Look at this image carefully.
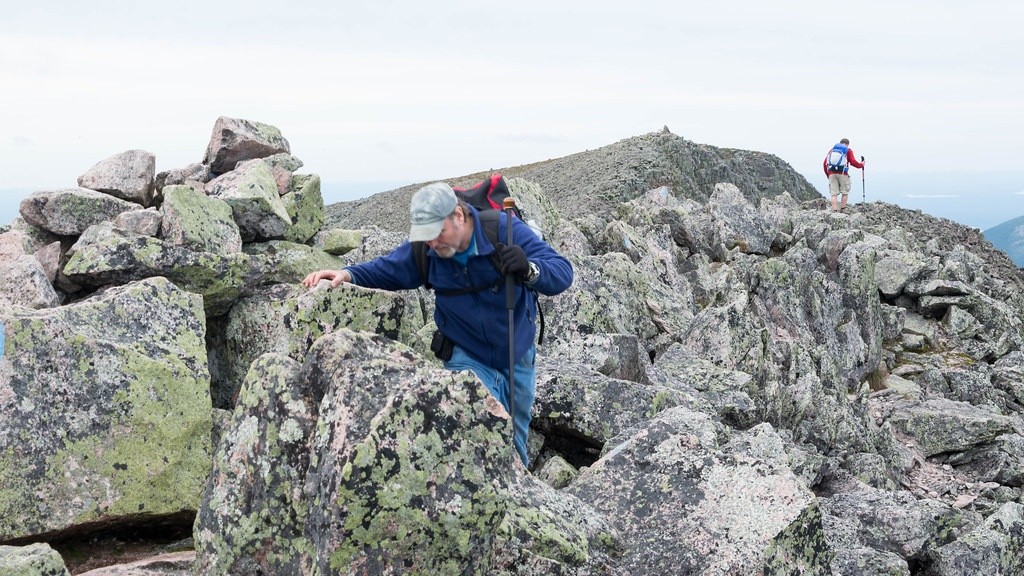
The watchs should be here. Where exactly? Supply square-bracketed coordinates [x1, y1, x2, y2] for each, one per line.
[529, 263, 540, 280]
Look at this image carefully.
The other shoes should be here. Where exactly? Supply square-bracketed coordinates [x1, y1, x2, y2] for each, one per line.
[840, 207, 851, 215]
[833, 209, 838, 213]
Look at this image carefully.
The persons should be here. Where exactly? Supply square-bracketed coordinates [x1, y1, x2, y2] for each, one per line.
[823, 138, 864, 212]
[303, 182, 574, 467]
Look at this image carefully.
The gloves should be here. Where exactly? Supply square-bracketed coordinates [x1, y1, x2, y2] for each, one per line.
[495, 242, 530, 280]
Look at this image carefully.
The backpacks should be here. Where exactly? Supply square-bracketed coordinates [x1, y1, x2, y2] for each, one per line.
[826, 142, 849, 172]
[411, 174, 526, 290]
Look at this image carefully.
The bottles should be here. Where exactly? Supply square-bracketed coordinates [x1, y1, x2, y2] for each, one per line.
[527, 219, 543, 242]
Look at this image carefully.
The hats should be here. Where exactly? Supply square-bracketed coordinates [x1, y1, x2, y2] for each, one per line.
[408, 183, 458, 242]
